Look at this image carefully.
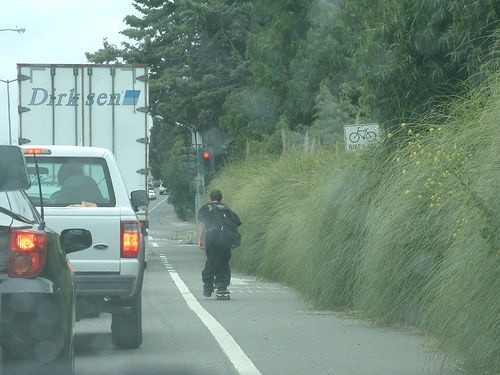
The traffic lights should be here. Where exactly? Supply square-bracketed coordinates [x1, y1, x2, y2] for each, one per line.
[198, 146, 214, 175]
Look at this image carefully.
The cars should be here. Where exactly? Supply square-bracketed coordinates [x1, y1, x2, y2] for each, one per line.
[148, 179, 168, 195]
[149, 190, 156, 200]
[0, 191, 93, 375]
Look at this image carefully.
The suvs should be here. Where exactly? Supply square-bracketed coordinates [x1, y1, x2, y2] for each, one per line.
[12, 144, 150, 350]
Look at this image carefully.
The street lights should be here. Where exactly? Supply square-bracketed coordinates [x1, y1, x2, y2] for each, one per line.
[0, 27, 27, 36]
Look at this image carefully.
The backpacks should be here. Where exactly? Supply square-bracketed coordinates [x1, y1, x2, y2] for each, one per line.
[207, 202, 241, 247]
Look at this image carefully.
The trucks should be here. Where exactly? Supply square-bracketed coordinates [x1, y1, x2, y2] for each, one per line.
[17, 62, 150, 239]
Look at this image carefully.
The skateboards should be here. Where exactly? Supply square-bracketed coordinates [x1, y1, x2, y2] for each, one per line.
[216, 290, 231, 301]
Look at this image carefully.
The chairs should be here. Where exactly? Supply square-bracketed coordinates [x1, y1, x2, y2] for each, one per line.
[55, 176, 104, 204]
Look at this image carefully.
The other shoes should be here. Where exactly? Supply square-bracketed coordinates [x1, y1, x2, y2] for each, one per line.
[216, 289, 230, 300]
[203, 284, 213, 297]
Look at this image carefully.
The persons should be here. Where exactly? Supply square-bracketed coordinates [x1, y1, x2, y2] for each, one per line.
[198, 189, 242, 301]
[46, 161, 85, 204]
